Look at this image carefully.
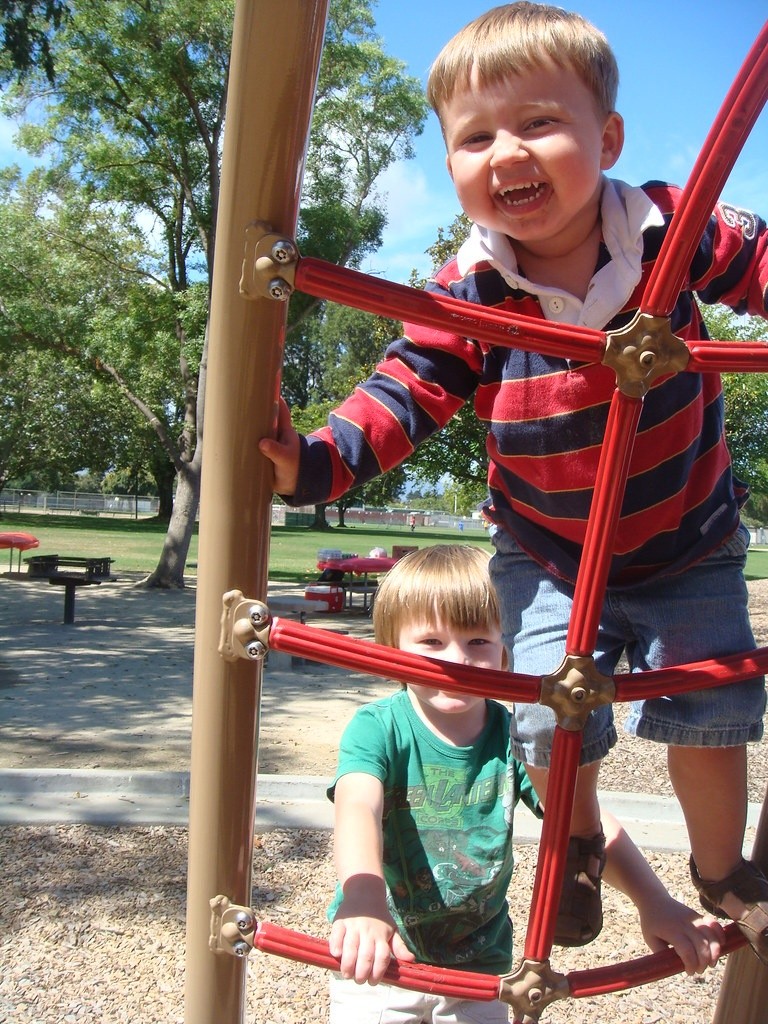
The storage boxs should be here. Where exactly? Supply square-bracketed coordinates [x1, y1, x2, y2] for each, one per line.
[305, 584, 344, 612]
[392, 545, 419, 560]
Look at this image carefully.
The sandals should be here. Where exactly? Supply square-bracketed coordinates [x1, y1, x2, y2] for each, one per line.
[690, 852, 768, 967]
[552, 825, 605, 946]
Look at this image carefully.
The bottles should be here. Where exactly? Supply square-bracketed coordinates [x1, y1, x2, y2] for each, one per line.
[317, 548, 343, 563]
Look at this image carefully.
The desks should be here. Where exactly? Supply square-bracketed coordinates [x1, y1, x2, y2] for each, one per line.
[266, 597, 330, 668]
[318, 558, 398, 614]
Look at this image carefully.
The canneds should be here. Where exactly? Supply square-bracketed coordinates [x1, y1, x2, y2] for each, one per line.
[342, 553, 358, 558]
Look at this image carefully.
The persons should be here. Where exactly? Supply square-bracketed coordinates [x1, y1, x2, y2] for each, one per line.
[458, 520, 464, 532]
[410, 516, 415, 531]
[483, 520, 489, 534]
[258, 0, 768, 966]
[325, 544, 727, 1024]
